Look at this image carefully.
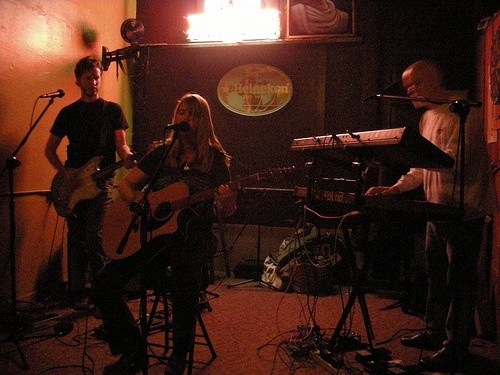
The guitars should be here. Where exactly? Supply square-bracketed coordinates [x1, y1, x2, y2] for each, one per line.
[51, 140, 164, 218]
[102, 165, 302, 261]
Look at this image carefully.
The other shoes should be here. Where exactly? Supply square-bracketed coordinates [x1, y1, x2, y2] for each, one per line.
[102, 349, 150, 375]
[164, 335, 187, 375]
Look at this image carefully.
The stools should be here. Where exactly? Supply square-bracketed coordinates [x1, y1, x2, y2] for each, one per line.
[147, 289, 217, 375]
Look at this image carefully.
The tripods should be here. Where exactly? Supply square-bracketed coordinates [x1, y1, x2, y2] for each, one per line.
[0, 100, 60, 370]
[227, 192, 278, 291]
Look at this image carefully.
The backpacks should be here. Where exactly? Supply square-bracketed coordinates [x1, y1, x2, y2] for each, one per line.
[261, 223, 348, 296]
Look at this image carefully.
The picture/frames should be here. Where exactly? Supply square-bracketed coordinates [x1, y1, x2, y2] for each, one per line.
[286, 0, 355, 39]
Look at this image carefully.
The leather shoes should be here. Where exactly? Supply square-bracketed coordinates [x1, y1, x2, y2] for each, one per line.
[400, 331, 452, 347]
[418, 345, 472, 371]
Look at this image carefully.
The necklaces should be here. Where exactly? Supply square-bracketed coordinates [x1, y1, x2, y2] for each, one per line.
[179, 153, 195, 171]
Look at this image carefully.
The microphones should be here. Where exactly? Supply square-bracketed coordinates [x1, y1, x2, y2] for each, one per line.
[164, 121, 189, 131]
[39, 89, 65, 99]
[363, 83, 401, 104]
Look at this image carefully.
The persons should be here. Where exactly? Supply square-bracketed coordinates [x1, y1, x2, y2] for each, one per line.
[45, 58, 139, 314]
[91, 93, 242, 375]
[289, 0, 349, 36]
[364, 61, 498, 364]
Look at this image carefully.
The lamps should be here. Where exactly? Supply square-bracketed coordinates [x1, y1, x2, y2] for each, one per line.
[102, 19, 155, 76]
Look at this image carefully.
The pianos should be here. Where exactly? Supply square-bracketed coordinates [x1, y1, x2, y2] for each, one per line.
[237, 126, 464, 375]
